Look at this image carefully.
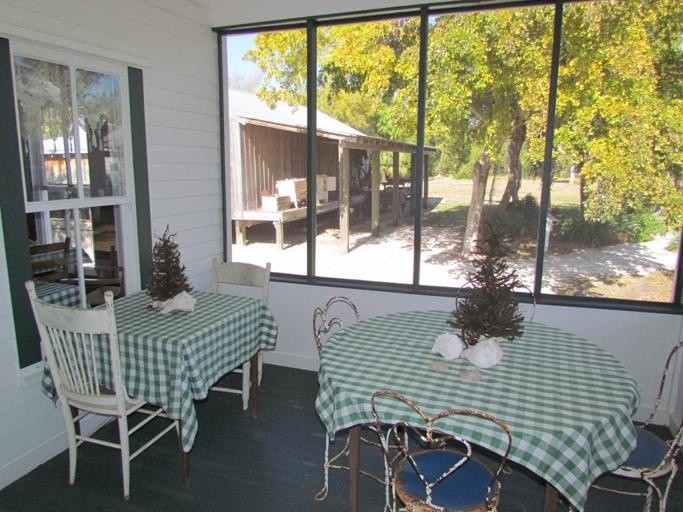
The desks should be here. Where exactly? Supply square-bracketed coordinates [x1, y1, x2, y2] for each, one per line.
[313, 310, 640, 512]
[30, 244, 92, 274]
[40, 289, 277, 488]
[35, 281, 81, 307]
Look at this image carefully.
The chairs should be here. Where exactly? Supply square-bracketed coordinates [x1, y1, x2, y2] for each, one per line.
[591, 341, 683, 511]
[25, 280, 180, 501]
[30, 237, 70, 282]
[86, 267, 124, 305]
[209, 257, 271, 411]
[313, 296, 407, 502]
[371, 389, 512, 511]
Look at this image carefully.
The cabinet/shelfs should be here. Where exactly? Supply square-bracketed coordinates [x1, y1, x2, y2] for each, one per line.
[276, 174, 337, 208]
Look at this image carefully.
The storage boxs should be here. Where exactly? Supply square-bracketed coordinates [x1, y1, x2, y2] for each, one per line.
[262, 194, 290, 211]
